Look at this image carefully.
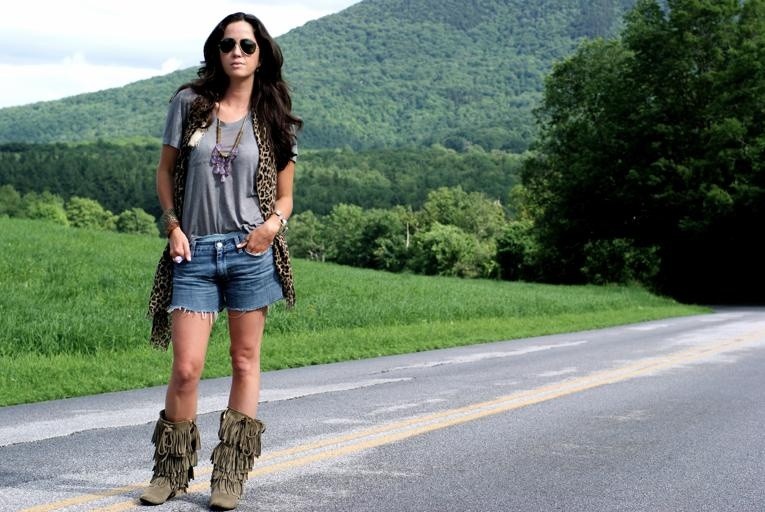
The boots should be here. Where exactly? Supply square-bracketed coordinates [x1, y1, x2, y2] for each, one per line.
[139, 408, 201, 507]
[207, 408, 264, 511]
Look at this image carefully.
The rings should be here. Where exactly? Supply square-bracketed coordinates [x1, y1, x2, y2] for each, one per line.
[174, 256, 182, 264]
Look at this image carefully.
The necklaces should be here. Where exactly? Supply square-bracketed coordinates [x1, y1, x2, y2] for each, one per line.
[209, 104, 246, 183]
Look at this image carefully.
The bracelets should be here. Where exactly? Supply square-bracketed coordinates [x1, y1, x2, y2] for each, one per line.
[274, 211, 288, 226]
[159, 208, 176, 237]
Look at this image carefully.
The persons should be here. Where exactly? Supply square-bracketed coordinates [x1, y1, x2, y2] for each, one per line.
[140, 10, 299, 511]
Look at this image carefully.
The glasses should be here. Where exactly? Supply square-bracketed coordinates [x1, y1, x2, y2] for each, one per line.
[218, 37, 258, 56]
